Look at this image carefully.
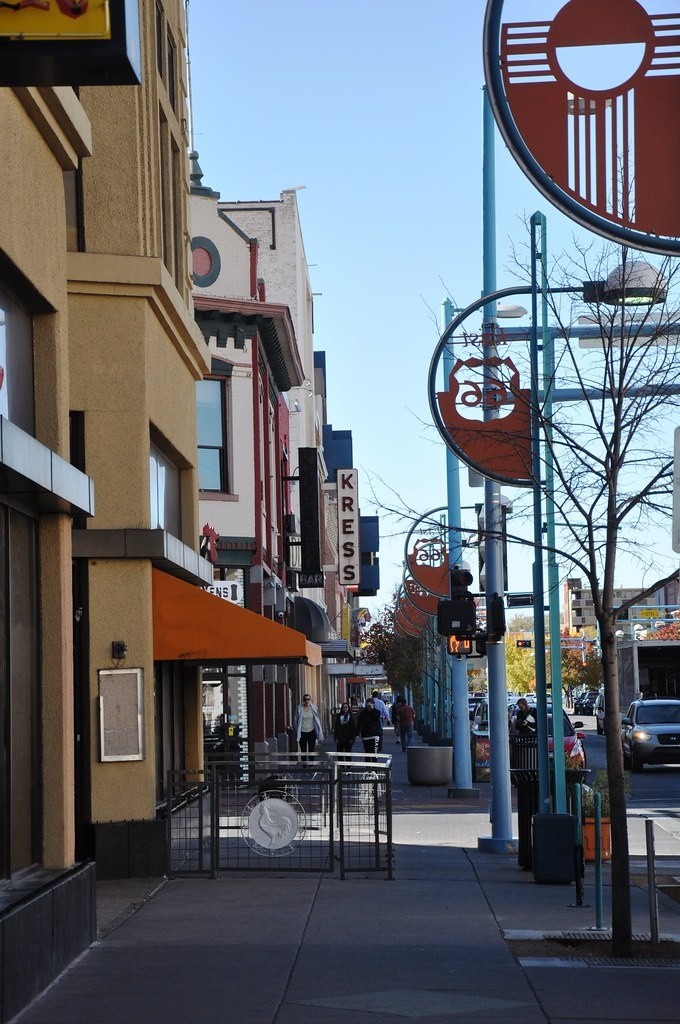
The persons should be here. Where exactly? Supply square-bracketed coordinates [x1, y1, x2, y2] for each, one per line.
[371, 691, 415, 752]
[516, 698, 537, 734]
[356, 698, 382, 773]
[334, 703, 356, 775]
[665, 608, 674, 628]
[294, 694, 324, 776]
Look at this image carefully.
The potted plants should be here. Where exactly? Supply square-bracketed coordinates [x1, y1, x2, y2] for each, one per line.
[556, 751, 631, 861]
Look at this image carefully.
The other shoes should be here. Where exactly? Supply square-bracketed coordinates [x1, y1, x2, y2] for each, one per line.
[396, 737, 400, 744]
[303, 767, 309, 776]
[402, 748, 407, 752]
[310, 767, 315, 777]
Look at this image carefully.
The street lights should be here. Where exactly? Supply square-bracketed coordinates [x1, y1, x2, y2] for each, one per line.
[404, 495, 515, 789]
[443, 298, 527, 794]
[427, 212, 669, 813]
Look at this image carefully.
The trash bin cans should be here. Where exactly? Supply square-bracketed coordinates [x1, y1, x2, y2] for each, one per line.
[509, 733, 538, 780]
[508, 768, 591, 869]
[531, 813, 576, 884]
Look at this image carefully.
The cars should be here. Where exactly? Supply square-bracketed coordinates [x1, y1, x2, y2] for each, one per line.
[621, 696, 680, 773]
[432, 686, 605, 785]
[382, 690, 393, 704]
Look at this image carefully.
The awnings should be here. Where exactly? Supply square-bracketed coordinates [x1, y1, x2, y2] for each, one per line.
[317, 640, 387, 684]
[295, 596, 332, 643]
[152, 566, 323, 668]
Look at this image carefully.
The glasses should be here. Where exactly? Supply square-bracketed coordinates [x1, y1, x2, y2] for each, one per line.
[365, 704, 372, 707]
[303, 698, 309, 701]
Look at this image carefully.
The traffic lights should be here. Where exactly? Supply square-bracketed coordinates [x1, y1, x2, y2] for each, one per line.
[516, 640, 532, 648]
[451, 570, 474, 604]
[446, 633, 473, 655]
